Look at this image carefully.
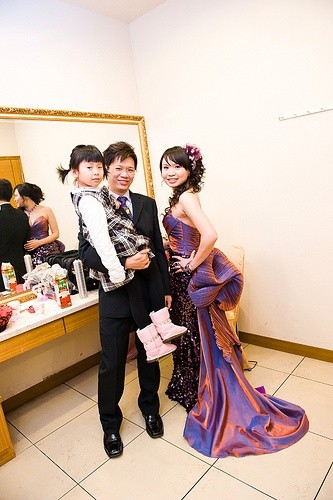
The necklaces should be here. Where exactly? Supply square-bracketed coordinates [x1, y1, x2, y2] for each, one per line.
[24, 204, 37, 212]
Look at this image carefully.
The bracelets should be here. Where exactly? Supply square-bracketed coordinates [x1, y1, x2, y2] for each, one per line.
[185, 261, 191, 272]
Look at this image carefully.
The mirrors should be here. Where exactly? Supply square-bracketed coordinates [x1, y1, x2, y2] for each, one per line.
[0, 107, 155, 307]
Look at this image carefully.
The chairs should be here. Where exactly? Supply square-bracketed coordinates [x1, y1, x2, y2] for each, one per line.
[225, 244, 244, 333]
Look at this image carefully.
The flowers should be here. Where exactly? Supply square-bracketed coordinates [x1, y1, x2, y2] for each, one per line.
[183, 143, 202, 170]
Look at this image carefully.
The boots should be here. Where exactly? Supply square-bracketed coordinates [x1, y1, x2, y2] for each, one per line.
[136, 323, 177, 362]
[150, 306, 187, 343]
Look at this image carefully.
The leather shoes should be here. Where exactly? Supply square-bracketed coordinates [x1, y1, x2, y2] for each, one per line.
[143, 412, 164, 438]
[103, 431, 123, 458]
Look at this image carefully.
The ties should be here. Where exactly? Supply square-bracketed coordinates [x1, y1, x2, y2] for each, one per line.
[117, 197, 133, 221]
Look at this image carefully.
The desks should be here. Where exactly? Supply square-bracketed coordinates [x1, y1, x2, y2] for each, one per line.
[0, 291, 99, 466]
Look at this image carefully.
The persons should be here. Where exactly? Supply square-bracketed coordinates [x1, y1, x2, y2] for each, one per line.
[0, 178, 32, 293]
[14, 182, 65, 268]
[58, 145, 188, 363]
[77, 141, 172, 458]
[160, 146, 309, 458]
